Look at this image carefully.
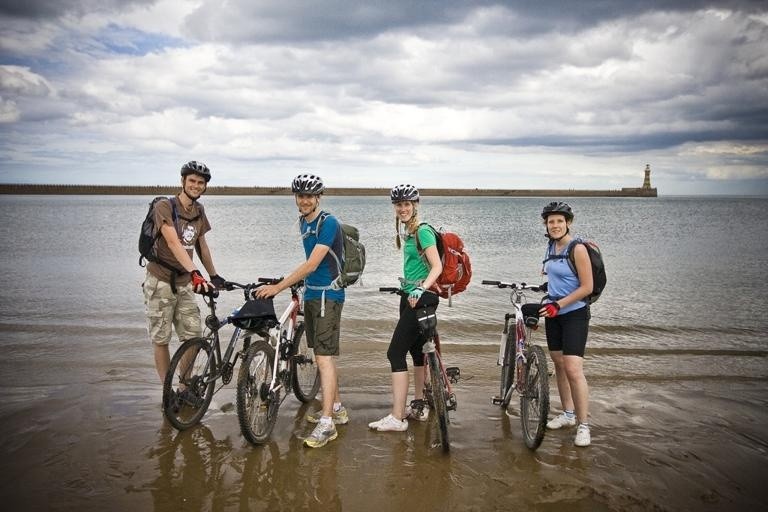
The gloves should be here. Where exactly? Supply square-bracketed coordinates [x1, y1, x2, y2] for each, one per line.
[191, 269, 217, 295]
[211, 274, 227, 292]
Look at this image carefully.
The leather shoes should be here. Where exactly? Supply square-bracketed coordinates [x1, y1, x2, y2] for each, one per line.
[170, 389, 180, 413]
[177, 387, 204, 409]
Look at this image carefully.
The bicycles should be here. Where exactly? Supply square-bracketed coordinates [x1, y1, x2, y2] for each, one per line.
[235, 279, 326, 446]
[380, 285, 460, 452]
[482, 279, 561, 451]
[162, 277, 281, 432]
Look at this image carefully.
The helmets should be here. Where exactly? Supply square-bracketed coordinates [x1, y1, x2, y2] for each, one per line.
[292, 174, 325, 196]
[180, 159, 210, 182]
[541, 201, 574, 221]
[390, 184, 419, 204]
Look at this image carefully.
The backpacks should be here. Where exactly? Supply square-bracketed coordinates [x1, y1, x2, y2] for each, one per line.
[137, 196, 177, 266]
[315, 212, 366, 291]
[416, 222, 472, 308]
[566, 238, 608, 307]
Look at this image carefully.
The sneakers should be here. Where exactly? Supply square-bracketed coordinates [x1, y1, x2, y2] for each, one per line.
[303, 417, 339, 449]
[306, 407, 348, 426]
[546, 412, 575, 430]
[574, 423, 590, 447]
[368, 413, 409, 433]
[404, 404, 430, 422]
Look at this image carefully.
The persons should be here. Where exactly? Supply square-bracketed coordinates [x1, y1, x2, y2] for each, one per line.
[539, 201, 594, 448]
[142, 159, 228, 412]
[367, 182, 443, 433]
[256, 174, 350, 450]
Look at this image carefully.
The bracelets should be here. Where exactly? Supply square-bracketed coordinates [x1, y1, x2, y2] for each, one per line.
[550, 299, 561, 313]
